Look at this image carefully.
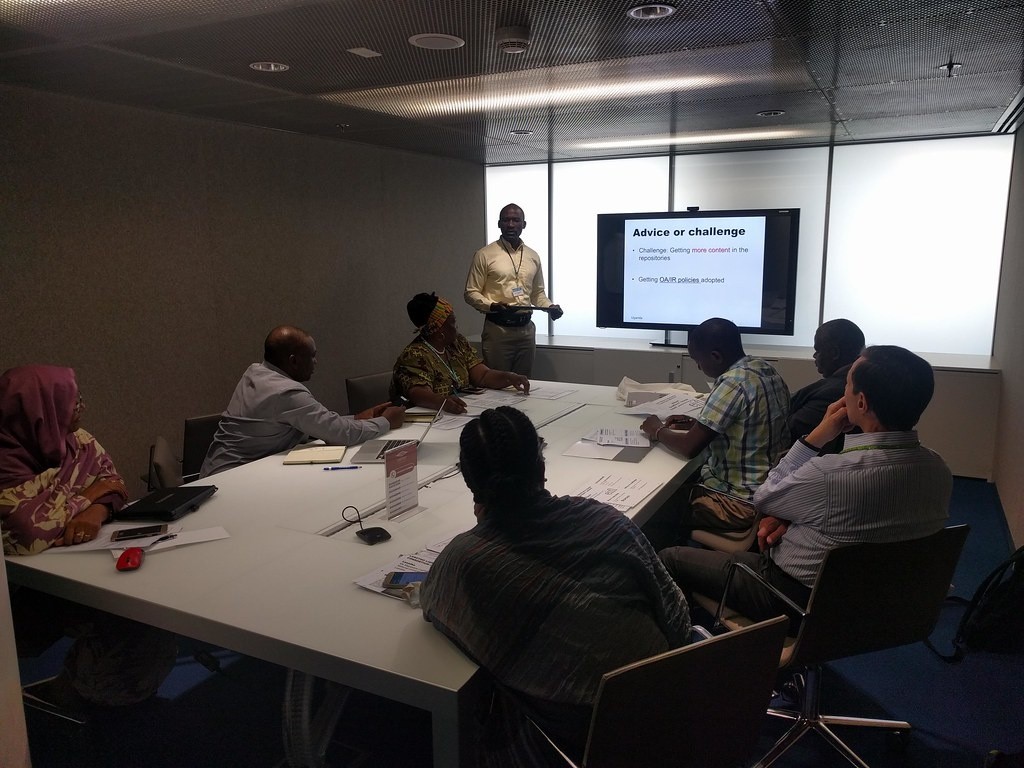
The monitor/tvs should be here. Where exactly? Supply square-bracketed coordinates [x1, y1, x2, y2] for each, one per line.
[596, 208, 799, 335]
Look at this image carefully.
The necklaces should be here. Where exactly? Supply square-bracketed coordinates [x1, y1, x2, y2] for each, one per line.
[421, 336, 446, 354]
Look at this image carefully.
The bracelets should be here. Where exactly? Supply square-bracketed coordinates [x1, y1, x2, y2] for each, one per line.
[655, 427, 665, 440]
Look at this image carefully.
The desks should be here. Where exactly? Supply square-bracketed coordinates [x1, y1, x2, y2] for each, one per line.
[5, 378, 713, 768]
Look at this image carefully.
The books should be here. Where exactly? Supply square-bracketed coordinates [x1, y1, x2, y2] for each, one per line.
[284, 444, 347, 463]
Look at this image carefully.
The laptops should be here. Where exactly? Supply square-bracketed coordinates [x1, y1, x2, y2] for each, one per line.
[350, 399, 447, 464]
[113, 485, 218, 521]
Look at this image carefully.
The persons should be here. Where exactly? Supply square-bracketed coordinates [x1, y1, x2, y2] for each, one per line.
[660, 347, 955, 708]
[791, 320, 864, 452]
[0, 368, 128, 717]
[388, 293, 530, 413]
[200, 325, 407, 477]
[640, 318, 793, 530]
[465, 203, 562, 379]
[420, 405, 694, 766]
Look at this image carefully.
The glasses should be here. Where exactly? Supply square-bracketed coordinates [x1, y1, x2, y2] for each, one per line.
[75, 390, 83, 414]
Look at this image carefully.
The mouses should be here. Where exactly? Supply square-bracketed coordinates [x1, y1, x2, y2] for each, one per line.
[115, 547, 144, 571]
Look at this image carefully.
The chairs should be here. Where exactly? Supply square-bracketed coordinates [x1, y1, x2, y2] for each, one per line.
[15, 413, 223, 726]
[520, 449, 970, 768]
[345, 371, 393, 415]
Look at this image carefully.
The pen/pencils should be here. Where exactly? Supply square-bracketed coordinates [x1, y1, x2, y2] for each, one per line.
[324, 466, 362, 471]
[669, 419, 693, 423]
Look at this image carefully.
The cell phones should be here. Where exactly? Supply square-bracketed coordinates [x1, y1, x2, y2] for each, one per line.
[382, 571, 429, 588]
[111, 523, 168, 542]
